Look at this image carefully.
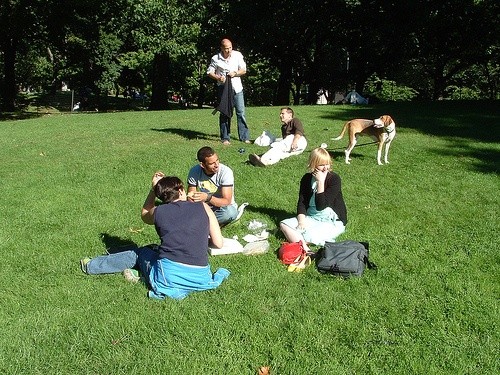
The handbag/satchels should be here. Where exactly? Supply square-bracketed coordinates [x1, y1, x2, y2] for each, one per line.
[310, 241, 377, 280]
[279, 240, 306, 264]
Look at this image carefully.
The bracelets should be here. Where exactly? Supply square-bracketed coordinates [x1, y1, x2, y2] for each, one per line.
[206, 193, 212, 202]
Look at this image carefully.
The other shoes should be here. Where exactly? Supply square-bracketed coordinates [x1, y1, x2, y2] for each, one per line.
[245, 140, 251, 144]
[222, 140, 230, 145]
[249, 154, 265, 168]
[123, 268, 142, 283]
[80, 257, 91, 273]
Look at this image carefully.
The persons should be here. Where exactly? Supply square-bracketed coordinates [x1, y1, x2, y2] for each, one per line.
[80, 173, 231, 299]
[280, 147, 348, 253]
[187, 147, 238, 226]
[123, 89, 127, 98]
[335, 98, 359, 105]
[207, 39, 250, 144]
[168, 92, 192, 108]
[249, 107, 307, 165]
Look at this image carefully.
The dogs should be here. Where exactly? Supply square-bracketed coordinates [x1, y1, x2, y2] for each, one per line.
[329, 115, 397, 166]
